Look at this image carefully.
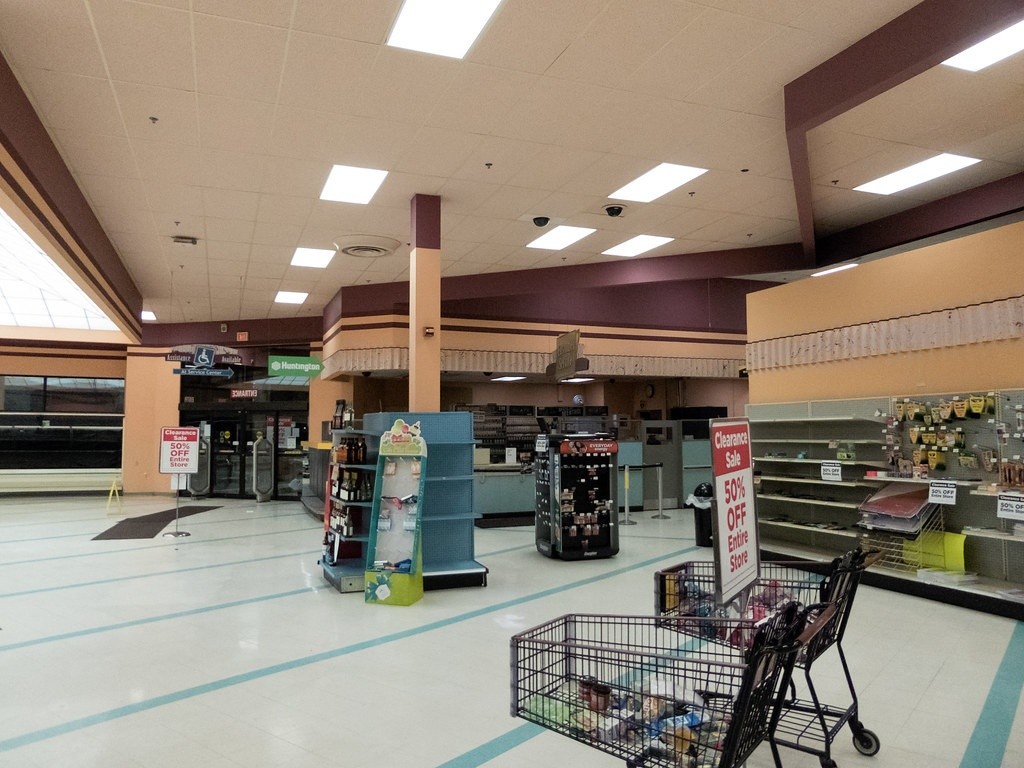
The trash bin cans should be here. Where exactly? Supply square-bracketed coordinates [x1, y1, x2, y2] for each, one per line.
[693, 482, 713, 547]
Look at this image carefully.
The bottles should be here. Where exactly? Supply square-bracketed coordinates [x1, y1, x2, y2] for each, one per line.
[329, 463, 372, 502]
[330, 501, 354, 537]
[332, 399, 355, 431]
[336, 434, 366, 464]
[322, 531, 335, 566]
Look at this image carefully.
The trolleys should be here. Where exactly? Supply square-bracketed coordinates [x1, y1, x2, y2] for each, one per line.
[511, 599, 842, 768]
[654, 543, 891, 768]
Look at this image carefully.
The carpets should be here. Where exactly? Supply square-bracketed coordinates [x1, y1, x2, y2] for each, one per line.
[90, 505, 224, 541]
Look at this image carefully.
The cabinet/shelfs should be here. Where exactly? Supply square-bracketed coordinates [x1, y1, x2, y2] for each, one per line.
[322, 412, 490, 593]
[744, 387, 1024, 621]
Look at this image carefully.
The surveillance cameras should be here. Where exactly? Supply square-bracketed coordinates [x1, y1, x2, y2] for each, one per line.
[606, 207, 622, 218]
[533, 217, 548, 227]
